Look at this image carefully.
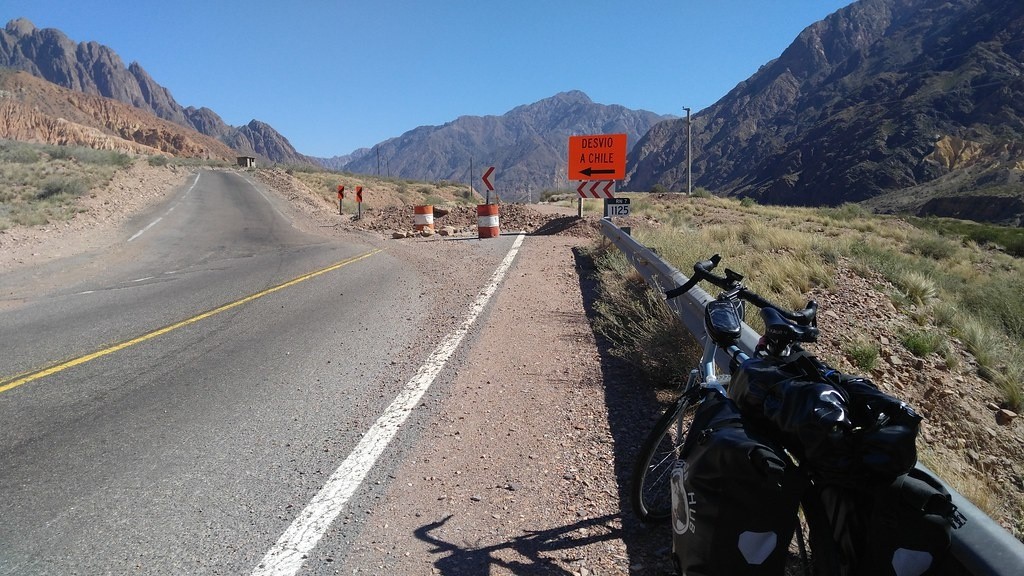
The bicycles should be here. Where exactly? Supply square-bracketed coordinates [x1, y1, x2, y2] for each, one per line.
[635, 254, 968, 576]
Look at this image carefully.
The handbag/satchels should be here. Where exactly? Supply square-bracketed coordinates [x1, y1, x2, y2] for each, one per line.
[672, 352, 968, 576]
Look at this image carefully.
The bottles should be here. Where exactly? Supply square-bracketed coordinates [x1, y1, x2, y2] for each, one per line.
[706, 375, 727, 397]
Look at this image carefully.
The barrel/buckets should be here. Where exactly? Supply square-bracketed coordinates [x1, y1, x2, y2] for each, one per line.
[477, 203, 499, 237]
[414, 206, 433, 233]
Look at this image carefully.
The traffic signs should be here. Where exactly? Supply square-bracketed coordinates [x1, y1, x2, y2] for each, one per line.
[567, 133, 625, 181]
